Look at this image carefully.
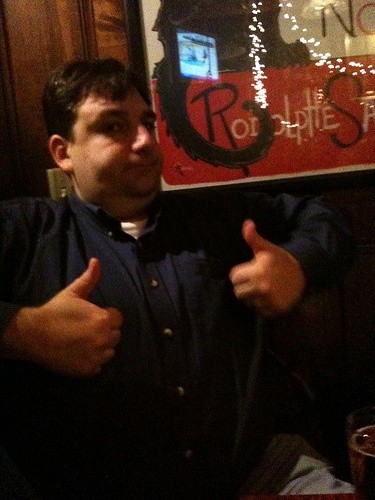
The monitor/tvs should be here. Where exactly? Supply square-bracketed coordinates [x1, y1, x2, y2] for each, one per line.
[174, 29, 218, 82]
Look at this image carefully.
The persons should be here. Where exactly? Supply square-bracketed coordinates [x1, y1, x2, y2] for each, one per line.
[0, 56, 357, 500]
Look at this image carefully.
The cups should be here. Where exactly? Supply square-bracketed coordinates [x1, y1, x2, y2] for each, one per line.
[347, 407, 375, 500]
